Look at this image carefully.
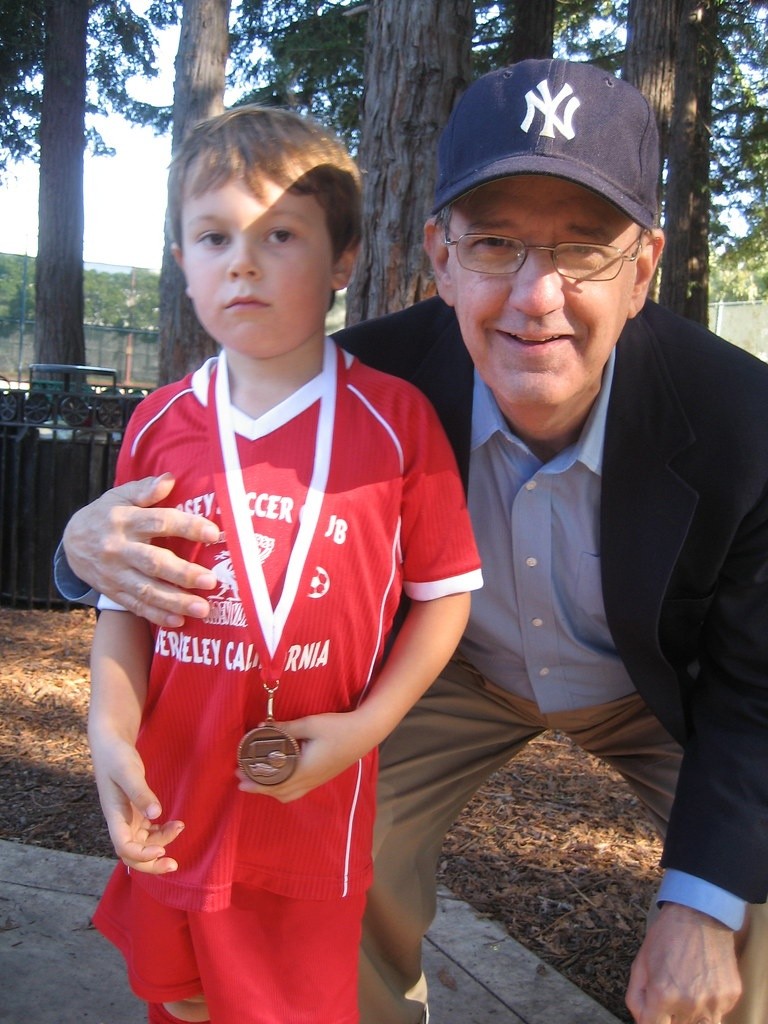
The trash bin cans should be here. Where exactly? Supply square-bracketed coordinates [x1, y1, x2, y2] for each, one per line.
[0, 389, 144, 612]
[28, 364, 118, 407]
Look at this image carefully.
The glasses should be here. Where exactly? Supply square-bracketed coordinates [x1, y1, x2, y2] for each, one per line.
[444, 227, 644, 282]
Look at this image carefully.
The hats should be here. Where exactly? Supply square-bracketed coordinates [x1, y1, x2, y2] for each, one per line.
[431, 59, 661, 231]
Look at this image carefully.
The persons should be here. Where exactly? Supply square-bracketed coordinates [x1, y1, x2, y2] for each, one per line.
[85, 102, 485, 1024]
[52, 58, 768, 1024]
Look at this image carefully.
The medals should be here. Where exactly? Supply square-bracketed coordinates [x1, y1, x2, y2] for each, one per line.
[238, 725, 301, 785]
